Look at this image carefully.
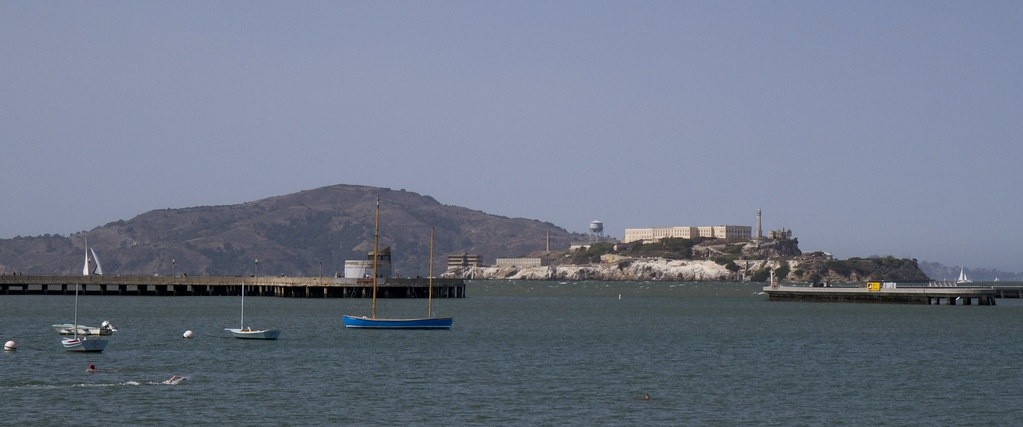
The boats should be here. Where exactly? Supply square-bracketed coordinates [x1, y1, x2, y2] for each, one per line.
[52, 324, 113, 335]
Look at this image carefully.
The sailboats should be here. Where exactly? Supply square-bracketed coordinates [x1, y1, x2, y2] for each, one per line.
[61, 282, 110, 352]
[224, 277, 291, 342]
[342, 195, 455, 329]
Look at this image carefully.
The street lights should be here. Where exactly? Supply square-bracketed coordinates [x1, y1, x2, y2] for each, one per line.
[254, 259, 259, 276]
[172, 260, 178, 277]
